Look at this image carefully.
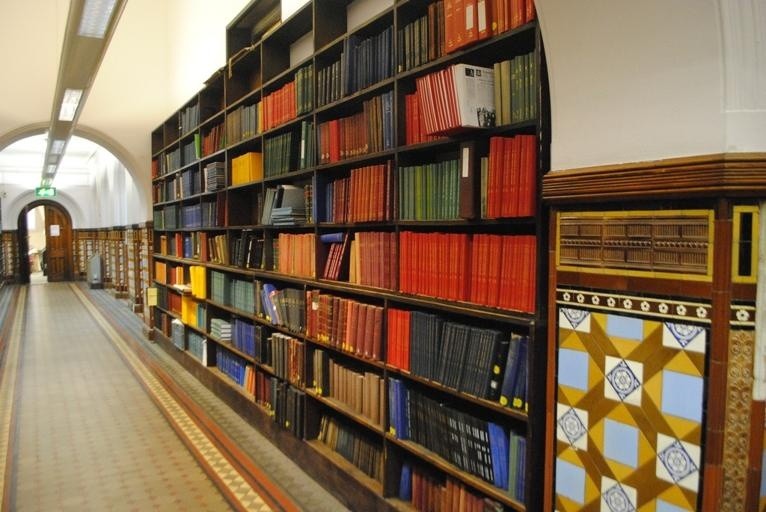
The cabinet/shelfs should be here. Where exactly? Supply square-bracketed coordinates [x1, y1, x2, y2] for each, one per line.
[149, 1, 552, 512]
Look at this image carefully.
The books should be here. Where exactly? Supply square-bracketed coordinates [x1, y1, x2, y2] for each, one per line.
[273, 232, 315, 279]
[307, 289, 384, 363]
[230, 231, 263, 269]
[187, 331, 208, 366]
[169, 234, 182, 257]
[154, 209, 165, 229]
[157, 152, 166, 175]
[184, 134, 200, 165]
[318, 231, 391, 291]
[388, 376, 527, 506]
[317, 26, 398, 106]
[168, 175, 179, 200]
[386, 305, 531, 414]
[398, 134, 536, 220]
[210, 271, 255, 315]
[399, 229, 538, 315]
[170, 266, 185, 289]
[184, 233, 199, 258]
[204, 162, 224, 192]
[231, 152, 263, 184]
[216, 344, 255, 397]
[169, 292, 179, 313]
[165, 149, 181, 173]
[199, 232, 227, 265]
[406, 50, 538, 145]
[171, 318, 185, 351]
[147, 284, 169, 308]
[312, 348, 384, 429]
[399, 462, 507, 512]
[156, 182, 168, 201]
[259, 282, 306, 333]
[172, 284, 192, 293]
[324, 161, 395, 223]
[201, 194, 223, 226]
[261, 184, 312, 223]
[180, 171, 200, 198]
[181, 295, 205, 333]
[180, 204, 200, 227]
[264, 64, 315, 132]
[189, 265, 209, 300]
[160, 235, 168, 256]
[264, 121, 314, 177]
[155, 262, 168, 286]
[202, 123, 226, 157]
[259, 372, 303, 440]
[399, 0, 537, 73]
[165, 205, 178, 228]
[154, 308, 168, 336]
[261, 333, 305, 389]
[316, 416, 386, 484]
[212, 317, 259, 358]
[316, 91, 399, 164]
[180, 104, 199, 136]
[227, 102, 263, 148]
